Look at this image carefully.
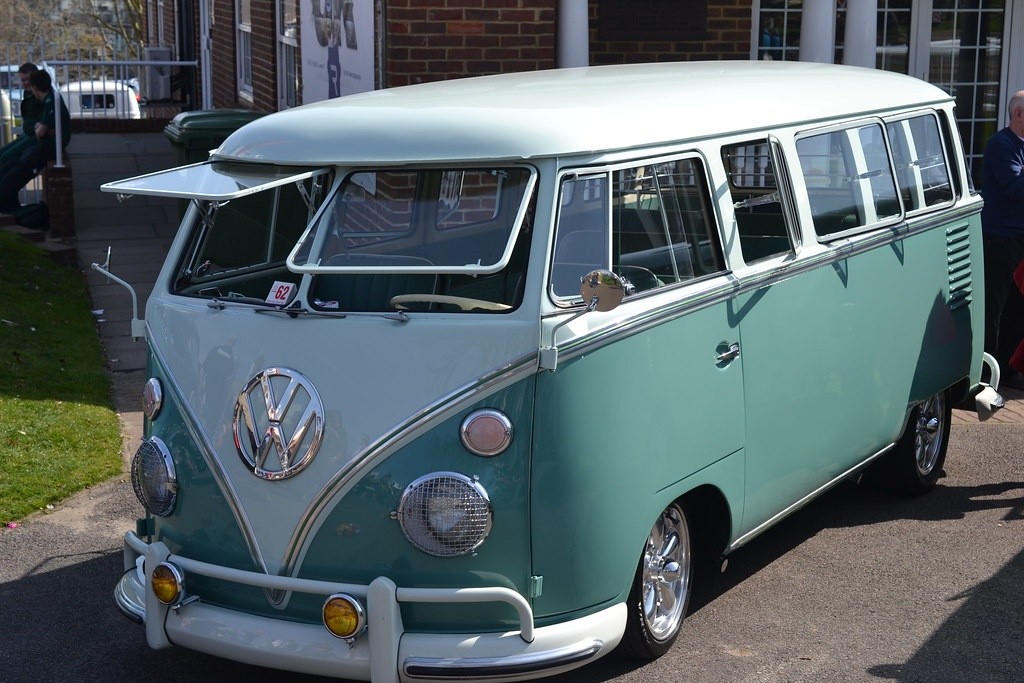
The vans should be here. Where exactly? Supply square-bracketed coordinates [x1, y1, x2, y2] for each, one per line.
[0, 65, 56, 149]
[88, 61, 1005, 681]
[58, 80, 140, 120]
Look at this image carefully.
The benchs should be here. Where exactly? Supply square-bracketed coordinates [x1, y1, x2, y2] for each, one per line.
[511, 194, 911, 310]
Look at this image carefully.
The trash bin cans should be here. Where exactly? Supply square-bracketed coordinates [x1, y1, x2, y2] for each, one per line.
[163, 109, 329, 271]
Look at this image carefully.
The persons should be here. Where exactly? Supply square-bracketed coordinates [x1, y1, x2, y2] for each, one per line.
[981, 90, 1024, 388]
[0, 63, 72, 216]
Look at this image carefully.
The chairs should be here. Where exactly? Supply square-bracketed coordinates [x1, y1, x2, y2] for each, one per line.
[307, 253, 438, 312]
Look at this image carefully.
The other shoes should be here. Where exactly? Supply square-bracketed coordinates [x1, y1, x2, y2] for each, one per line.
[1000, 372, 1024, 390]
